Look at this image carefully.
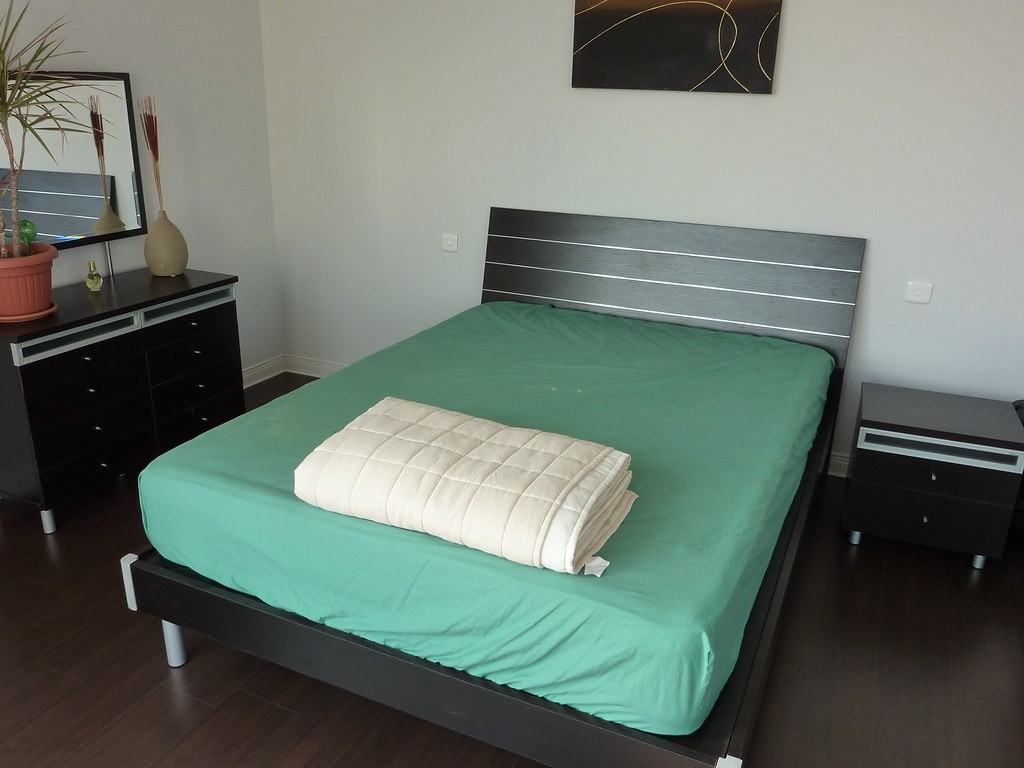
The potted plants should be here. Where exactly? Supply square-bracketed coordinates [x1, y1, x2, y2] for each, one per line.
[0, 0, 127, 325]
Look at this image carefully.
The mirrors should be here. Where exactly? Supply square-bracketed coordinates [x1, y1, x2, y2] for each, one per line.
[0, 70, 148, 250]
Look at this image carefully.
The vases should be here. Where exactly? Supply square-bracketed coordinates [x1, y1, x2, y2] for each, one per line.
[93, 199, 125, 231]
[145, 210, 189, 278]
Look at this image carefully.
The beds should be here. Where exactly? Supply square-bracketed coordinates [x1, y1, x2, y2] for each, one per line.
[121, 206, 867, 768]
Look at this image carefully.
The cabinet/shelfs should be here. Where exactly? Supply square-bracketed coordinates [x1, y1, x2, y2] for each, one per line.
[840, 382, 1024, 570]
[0, 268, 246, 535]
